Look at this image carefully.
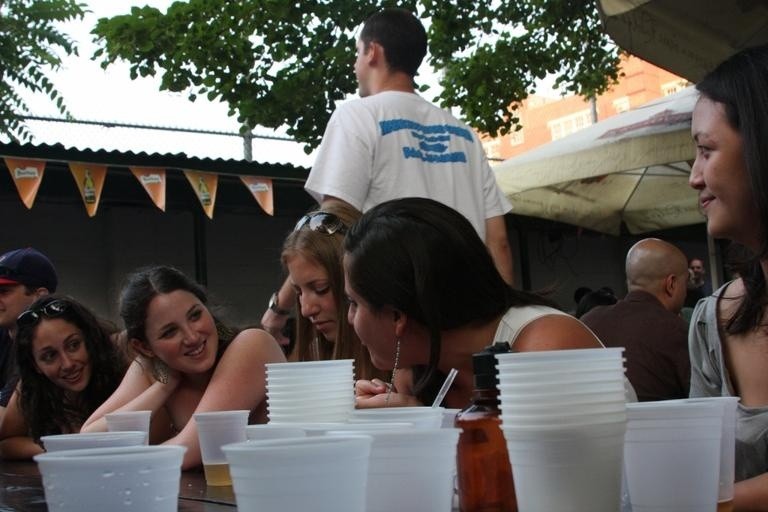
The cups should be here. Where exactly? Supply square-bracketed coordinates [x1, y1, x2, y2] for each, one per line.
[35, 430, 149, 454]
[32, 446, 189, 511]
[626, 390, 740, 511]
[102, 409, 153, 445]
[261, 354, 358, 423]
[191, 408, 253, 489]
[490, 342, 627, 512]
[228, 405, 460, 512]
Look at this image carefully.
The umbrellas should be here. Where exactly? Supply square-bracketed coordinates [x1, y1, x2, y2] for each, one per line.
[479, 83, 726, 295]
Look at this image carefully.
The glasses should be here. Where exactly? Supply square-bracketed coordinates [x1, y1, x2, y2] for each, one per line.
[17, 300, 67, 329]
[293, 212, 348, 240]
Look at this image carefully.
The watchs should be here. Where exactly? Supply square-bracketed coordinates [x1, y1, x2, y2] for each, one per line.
[267, 292, 292, 318]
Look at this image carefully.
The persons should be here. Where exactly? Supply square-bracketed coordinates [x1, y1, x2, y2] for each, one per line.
[576, 235, 689, 397]
[0, 293, 140, 463]
[281, 208, 394, 383]
[686, 258, 712, 297]
[682, 47, 767, 510]
[79, 262, 292, 471]
[0, 245, 60, 432]
[260, 0, 516, 347]
[339, 196, 640, 512]
[576, 290, 619, 318]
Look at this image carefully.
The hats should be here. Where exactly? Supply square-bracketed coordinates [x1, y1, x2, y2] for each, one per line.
[0, 248, 57, 292]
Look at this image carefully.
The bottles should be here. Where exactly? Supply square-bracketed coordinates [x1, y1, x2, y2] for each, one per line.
[455, 340, 518, 512]
